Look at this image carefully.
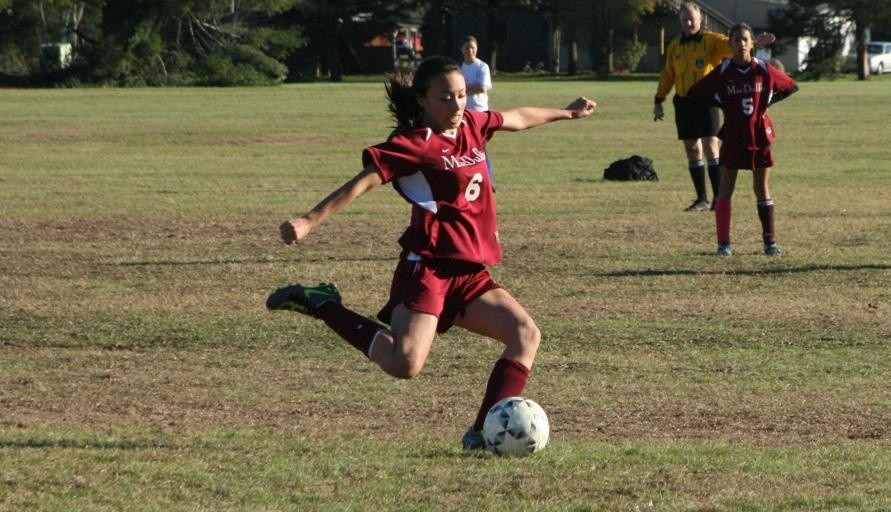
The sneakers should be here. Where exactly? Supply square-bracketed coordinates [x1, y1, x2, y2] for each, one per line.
[717, 245, 732, 256]
[266, 283, 343, 320]
[763, 245, 782, 257]
[461, 426, 485, 450]
[685, 200, 711, 212]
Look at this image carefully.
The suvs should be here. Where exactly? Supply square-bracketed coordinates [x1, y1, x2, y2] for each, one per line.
[842, 41, 891, 75]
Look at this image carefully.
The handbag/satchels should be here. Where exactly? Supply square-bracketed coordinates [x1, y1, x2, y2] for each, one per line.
[604, 155, 658, 181]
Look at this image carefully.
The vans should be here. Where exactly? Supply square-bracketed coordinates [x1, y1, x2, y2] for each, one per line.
[39, 41, 72, 72]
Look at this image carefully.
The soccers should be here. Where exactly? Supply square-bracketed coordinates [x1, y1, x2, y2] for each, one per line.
[482, 396, 549, 458]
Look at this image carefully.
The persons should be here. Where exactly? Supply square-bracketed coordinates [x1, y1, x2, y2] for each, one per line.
[652, 1, 775, 209]
[460, 33, 496, 196]
[266, 57, 598, 449]
[687, 22, 799, 258]
